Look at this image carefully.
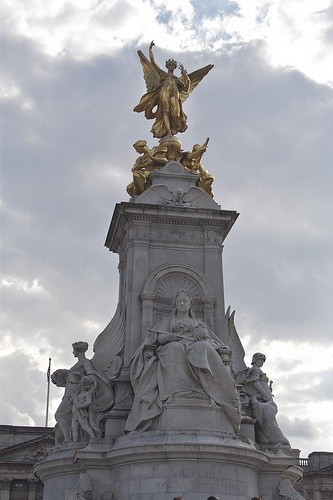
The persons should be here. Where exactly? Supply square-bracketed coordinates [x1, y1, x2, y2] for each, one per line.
[54, 341, 97, 443]
[148, 40, 190, 139]
[122, 290, 242, 435]
[241, 352, 290, 446]
[187, 142, 215, 198]
[126, 138, 168, 197]
